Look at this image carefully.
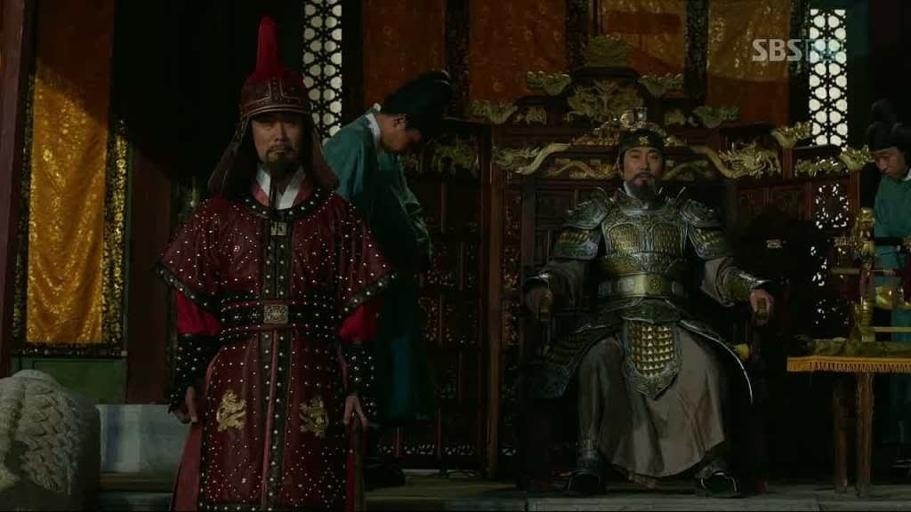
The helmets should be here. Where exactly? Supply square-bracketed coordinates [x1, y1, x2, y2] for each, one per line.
[237, 67, 313, 122]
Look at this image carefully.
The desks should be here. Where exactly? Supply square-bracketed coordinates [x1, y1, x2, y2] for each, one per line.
[786, 338, 911, 499]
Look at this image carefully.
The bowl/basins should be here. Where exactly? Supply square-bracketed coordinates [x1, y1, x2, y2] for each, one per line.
[814, 337, 846, 355]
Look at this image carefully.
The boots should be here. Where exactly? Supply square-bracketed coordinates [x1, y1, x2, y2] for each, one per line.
[882, 442, 910, 484]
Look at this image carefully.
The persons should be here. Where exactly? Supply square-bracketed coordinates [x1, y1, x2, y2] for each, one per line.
[323, 64, 459, 429]
[155, 15, 402, 509]
[861, 94, 911, 483]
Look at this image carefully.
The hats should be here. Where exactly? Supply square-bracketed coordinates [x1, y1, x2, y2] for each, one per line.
[619, 125, 665, 154]
[382, 78, 454, 129]
[867, 99, 911, 151]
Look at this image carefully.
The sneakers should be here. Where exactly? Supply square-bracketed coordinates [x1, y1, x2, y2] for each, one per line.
[694, 468, 740, 498]
[566, 466, 606, 498]
